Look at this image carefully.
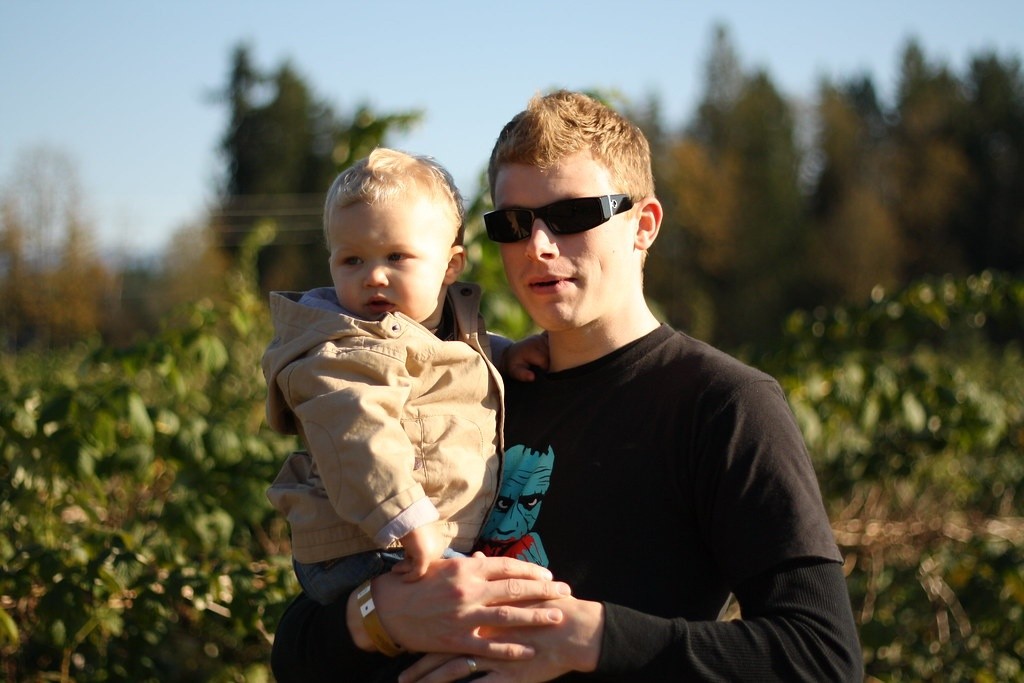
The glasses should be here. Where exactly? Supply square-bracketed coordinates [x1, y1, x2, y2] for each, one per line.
[484, 193, 646, 243]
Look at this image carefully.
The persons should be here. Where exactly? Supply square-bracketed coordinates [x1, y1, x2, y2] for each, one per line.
[262, 86, 864, 683]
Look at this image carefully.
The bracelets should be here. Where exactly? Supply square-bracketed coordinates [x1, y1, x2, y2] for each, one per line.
[353, 576, 406, 659]
